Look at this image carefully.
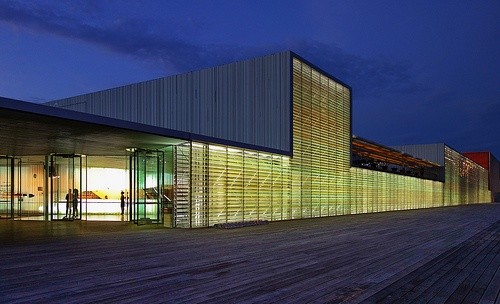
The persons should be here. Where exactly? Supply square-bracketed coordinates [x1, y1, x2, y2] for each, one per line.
[119, 191, 125, 215]
[105, 196, 108, 199]
[63, 189, 74, 220]
[72, 189, 79, 219]
